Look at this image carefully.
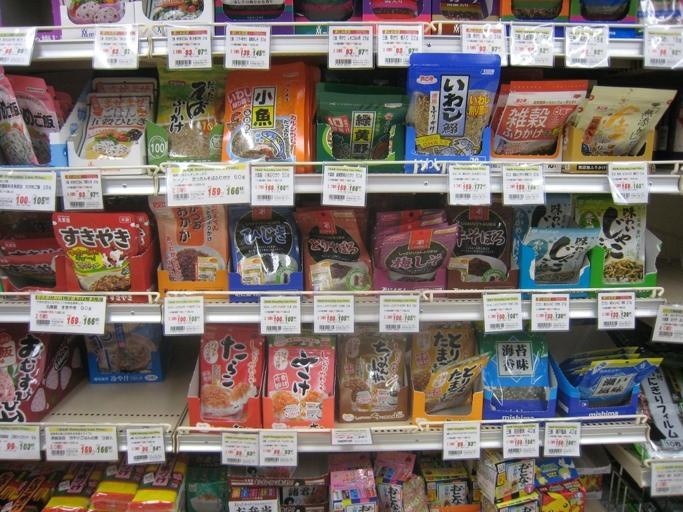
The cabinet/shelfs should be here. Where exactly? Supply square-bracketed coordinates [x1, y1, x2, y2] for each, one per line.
[0, 23, 683, 512]
